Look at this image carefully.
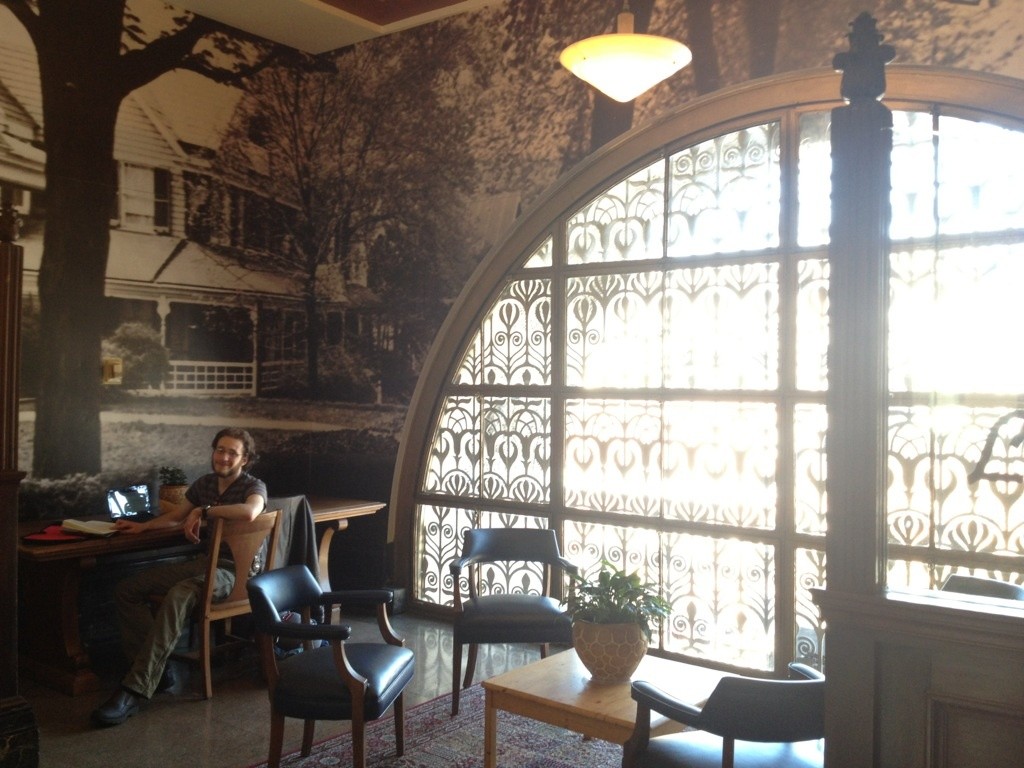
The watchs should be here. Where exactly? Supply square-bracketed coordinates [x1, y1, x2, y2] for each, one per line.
[201, 504, 211, 521]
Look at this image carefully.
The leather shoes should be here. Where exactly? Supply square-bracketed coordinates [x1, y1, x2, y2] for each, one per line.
[159, 662, 175, 692]
[91, 687, 137, 724]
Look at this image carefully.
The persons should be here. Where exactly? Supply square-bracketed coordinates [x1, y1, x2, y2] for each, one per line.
[92, 426, 266, 728]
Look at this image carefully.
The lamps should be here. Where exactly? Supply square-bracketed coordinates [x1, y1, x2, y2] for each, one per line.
[560, 0, 692, 103]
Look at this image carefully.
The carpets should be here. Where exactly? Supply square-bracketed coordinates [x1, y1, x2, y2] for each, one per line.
[241, 682, 626, 768]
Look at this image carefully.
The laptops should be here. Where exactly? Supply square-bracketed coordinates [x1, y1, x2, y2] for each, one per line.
[105, 481, 159, 523]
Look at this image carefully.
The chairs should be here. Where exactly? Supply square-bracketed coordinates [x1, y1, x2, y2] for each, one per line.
[449, 528, 577, 714]
[246, 563, 416, 768]
[940, 574, 1024, 602]
[149, 509, 282, 698]
[622, 662, 826, 768]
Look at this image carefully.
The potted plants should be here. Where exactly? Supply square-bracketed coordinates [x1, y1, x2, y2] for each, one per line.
[157, 465, 187, 506]
[556, 556, 675, 684]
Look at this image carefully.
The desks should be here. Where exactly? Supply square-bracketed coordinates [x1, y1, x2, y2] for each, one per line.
[480, 645, 741, 768]
[18, 496, 386, 692]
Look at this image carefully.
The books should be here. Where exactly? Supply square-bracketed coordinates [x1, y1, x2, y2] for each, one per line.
[61, 520, 122, 538]
